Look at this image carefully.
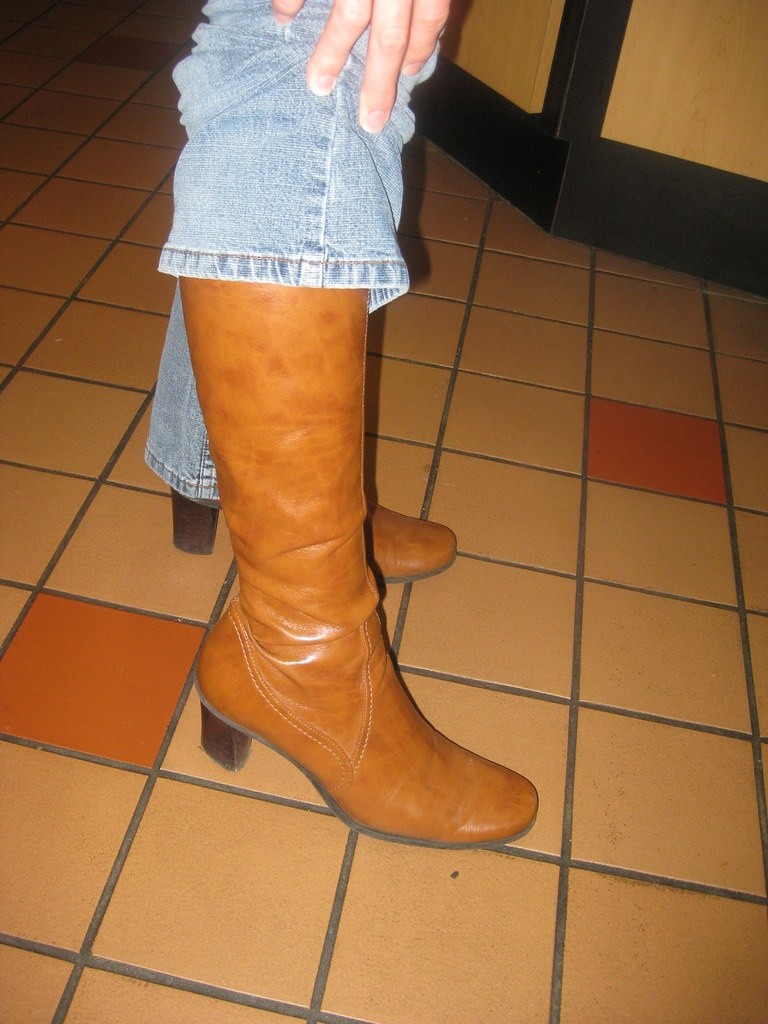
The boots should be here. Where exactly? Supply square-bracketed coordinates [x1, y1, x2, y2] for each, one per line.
[171, 474, 457, 584]
[176, 277, 539, 850]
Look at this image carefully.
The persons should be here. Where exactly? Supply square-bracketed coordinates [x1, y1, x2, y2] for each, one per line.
[146, 0, 539, 849]
